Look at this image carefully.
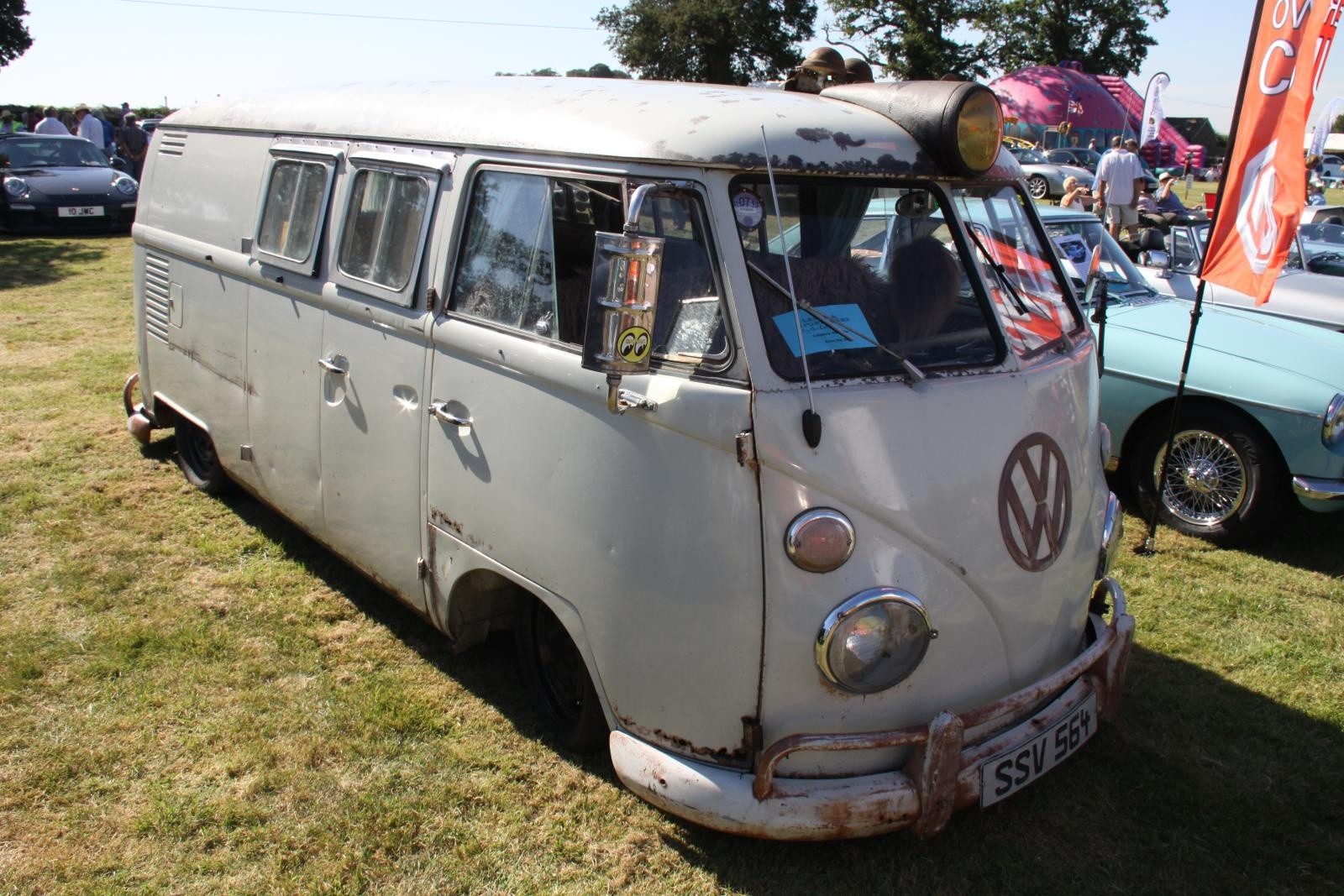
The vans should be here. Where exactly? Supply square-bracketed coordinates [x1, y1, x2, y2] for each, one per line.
[123, 50, 1134, 842]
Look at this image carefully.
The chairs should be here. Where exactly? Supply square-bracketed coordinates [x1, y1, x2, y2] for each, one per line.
[1140, 229, 1164, 251]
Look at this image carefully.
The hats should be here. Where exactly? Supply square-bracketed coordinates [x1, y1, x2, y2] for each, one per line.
[1159, 172, 1173, 182]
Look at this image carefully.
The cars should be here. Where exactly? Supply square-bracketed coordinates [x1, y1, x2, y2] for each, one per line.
[135, 119, 163, 143]
[1312, 154, 1344, 184]
[1176, 205, 1344, 277]
[1007, 147, 1095, 199]
[1099, 209, 1344, 337]
[1043, 147, 1102, 175]
[0, 132, 140, 241]
[752, 197, 1344, 538]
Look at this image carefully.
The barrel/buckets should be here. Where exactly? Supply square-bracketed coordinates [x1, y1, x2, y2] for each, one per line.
[1204, 192, 1217, 219]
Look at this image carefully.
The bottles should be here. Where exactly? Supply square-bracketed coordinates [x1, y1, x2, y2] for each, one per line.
[1167, 180, 1179, 185]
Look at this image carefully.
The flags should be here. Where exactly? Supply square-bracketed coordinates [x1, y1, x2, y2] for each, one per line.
[1068, 100, 1084, 115]
[1307, 98, 1344, 156]
[1199, 0, 1344, 308]
[1139, 72, 1170, 148]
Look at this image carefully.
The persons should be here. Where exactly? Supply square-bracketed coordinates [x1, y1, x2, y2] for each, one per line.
[1088, 138, 1096, 150]
[1090, 136, 1188, 242]
[1031, 141, 1043, 152]
[1184, 157, 1191, 175]
[1199, 163, 1223, 182]
[1306, 178, 1326, 206]
[0, 110, 24, 132]
[1060, 176, 1100, 211]
[25, 102, 150, 182]
